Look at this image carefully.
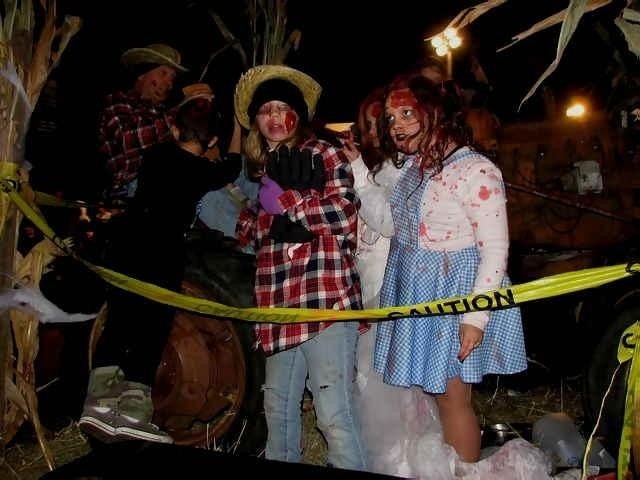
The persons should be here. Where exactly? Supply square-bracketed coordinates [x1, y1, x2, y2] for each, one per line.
[6, 44, 259, 445]
[234, 63, 528, 471]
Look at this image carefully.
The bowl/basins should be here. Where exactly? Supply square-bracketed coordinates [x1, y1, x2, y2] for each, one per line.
[83, 279, 250, 449]
[489, 423, 533, 445]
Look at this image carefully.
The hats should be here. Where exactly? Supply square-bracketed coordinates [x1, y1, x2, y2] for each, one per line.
[123, 44, 188, 72]
[235, 64, 323, 130]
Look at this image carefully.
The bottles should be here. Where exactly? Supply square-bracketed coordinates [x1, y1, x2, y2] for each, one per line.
[532, 411, 587, 467]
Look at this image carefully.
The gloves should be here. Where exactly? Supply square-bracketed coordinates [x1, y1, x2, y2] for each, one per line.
[259, 174, 286, 216]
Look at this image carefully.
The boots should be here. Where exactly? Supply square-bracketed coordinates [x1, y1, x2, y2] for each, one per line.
[76, 366, 176, 446]
